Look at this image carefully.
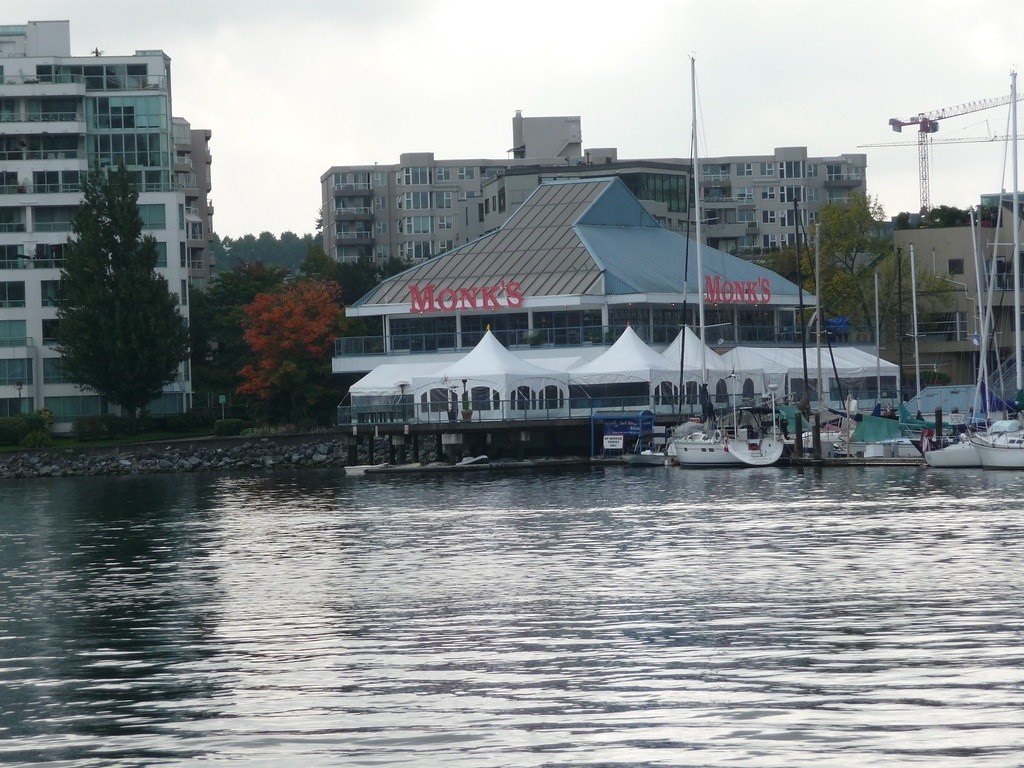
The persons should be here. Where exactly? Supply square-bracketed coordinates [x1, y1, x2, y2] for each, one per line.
[915, 411, 925, 421]
[844, 393, 859, 412]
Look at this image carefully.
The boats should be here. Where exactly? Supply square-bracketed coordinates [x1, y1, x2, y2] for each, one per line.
[342, 455, 489, 475]
[588, 56, 1024, 473]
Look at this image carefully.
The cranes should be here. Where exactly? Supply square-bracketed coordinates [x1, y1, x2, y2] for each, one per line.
[849, 91, 1024, 222]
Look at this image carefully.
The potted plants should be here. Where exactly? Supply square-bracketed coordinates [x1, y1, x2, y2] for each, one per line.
[461, 408, 473, 421]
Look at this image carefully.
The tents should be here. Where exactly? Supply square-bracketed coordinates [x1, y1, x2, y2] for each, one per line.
[411, 329, 571, 420]
[720, 346, 901, 410]
[347, 356, 589, 423]
[568, 323, 766, 415]
